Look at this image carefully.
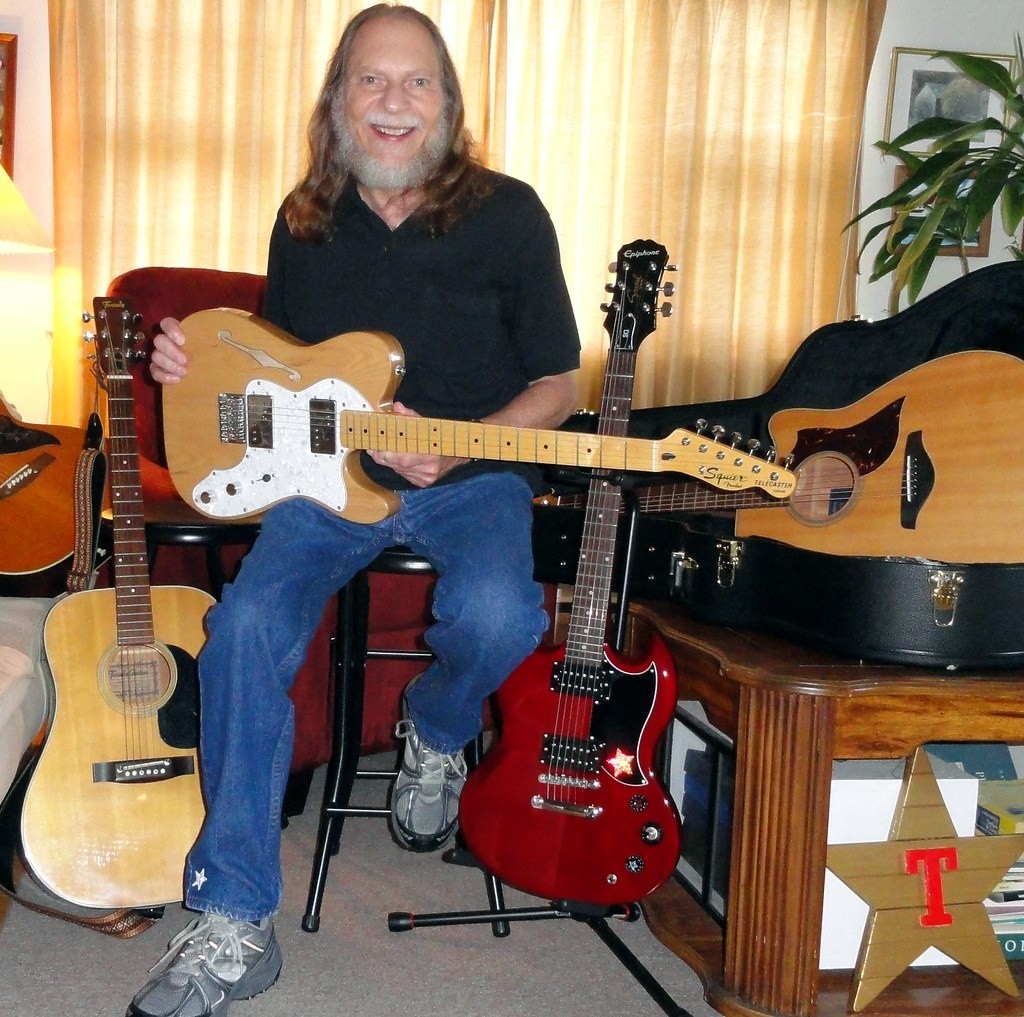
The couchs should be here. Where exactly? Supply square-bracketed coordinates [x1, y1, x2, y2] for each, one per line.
[87, 256, 562, 817]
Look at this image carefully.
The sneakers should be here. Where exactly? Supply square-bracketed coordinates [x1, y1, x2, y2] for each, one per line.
[391, 671, 469, 852]
[122, 906, 282, 1017]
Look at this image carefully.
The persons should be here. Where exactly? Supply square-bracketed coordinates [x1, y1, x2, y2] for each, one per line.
[128, 6, 580, 1017]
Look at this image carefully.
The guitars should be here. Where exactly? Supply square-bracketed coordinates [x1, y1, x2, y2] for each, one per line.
[0, 388, 107, 597]
[14, 295, 223, 911]
[157, 307, 802, 530]
[454, 235, 687, 913]
[531, 344, 1023, 568]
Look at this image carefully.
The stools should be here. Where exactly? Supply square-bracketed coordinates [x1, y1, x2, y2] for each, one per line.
[98, 487, 313, 820]
[303, 547, 515, 945]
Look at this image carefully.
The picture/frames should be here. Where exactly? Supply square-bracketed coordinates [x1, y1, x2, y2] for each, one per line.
[882, 46, 1016, 158]
[889, 164, 992, 258]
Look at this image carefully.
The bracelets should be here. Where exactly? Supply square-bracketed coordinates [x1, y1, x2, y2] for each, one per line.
[471, 421, 485, 462]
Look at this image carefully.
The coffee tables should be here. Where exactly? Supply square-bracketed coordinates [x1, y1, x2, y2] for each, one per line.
[552, 584, 1024, 1017]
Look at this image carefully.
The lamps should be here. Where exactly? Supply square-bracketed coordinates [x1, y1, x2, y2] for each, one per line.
[0, 150, 59, 260]
